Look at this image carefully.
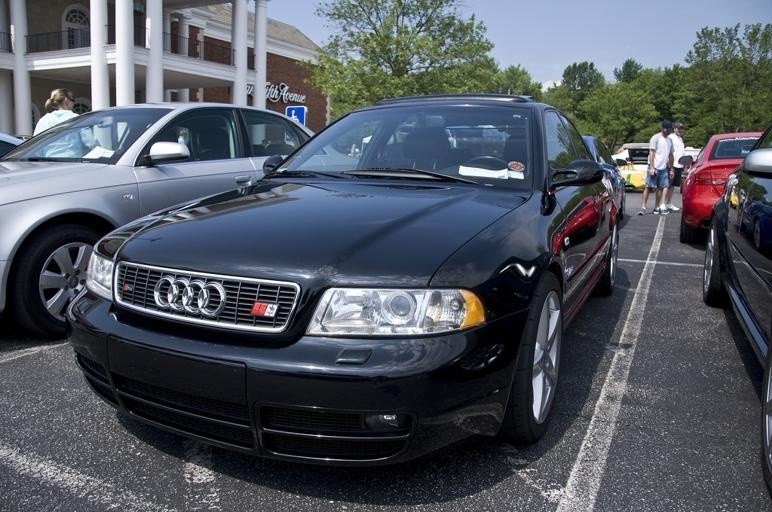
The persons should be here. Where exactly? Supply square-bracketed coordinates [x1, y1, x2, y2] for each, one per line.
[32, 88, 101, 158]
[636, 121, 685, 215]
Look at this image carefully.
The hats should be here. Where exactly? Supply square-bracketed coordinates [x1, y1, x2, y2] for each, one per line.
[674, 123, 686, 129]
[662, 120, 672, 128]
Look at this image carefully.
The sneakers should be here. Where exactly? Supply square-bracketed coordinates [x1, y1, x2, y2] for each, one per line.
[652, 204, 680, 214]
[636, 208, 647, 215]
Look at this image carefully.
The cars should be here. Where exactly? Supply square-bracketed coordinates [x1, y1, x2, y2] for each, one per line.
[72, 92, 622, 468]
[613, 141, 703, 192]
[581, 135, 632, 216]
[678, 132, 766, 246]
[0, 103, 364, 341]
[701, 124, 772, 488]
[0, 132, 27, 158]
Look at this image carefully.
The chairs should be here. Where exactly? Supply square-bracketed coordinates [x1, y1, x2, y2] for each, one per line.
[385, 128, 528, 171]
[196, 128, 229, 161]
